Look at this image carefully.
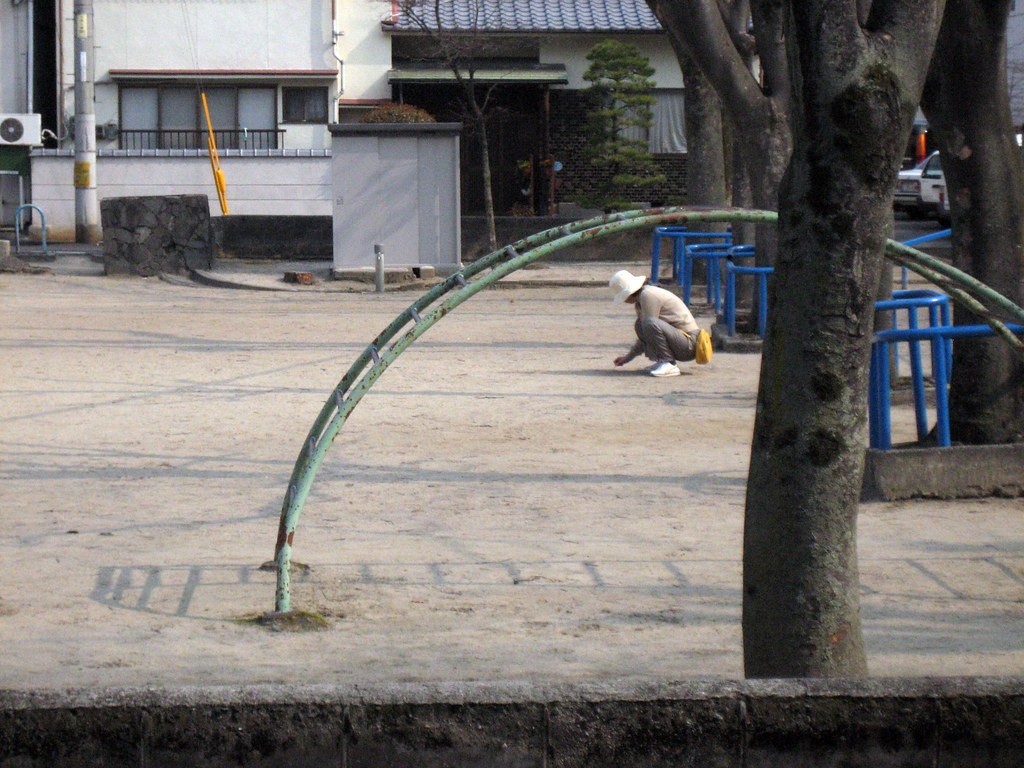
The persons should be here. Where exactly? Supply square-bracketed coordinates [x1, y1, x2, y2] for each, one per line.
[520, 162, 533, 210]
[608, 270, 700, 378]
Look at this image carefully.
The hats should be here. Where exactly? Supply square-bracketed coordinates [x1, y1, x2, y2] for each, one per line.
[609, 270, 646, 307]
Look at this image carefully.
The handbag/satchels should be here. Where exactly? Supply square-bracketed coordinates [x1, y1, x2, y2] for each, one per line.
[695, 328, 712, 364]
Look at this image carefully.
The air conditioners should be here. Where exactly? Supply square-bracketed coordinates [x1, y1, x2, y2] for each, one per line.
[0, 112, 42, 147]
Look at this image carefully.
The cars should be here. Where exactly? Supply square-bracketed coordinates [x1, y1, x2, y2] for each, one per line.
[891, 149, 951, 227]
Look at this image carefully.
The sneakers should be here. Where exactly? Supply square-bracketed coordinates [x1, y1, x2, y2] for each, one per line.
[645, 359, 680, 377]
